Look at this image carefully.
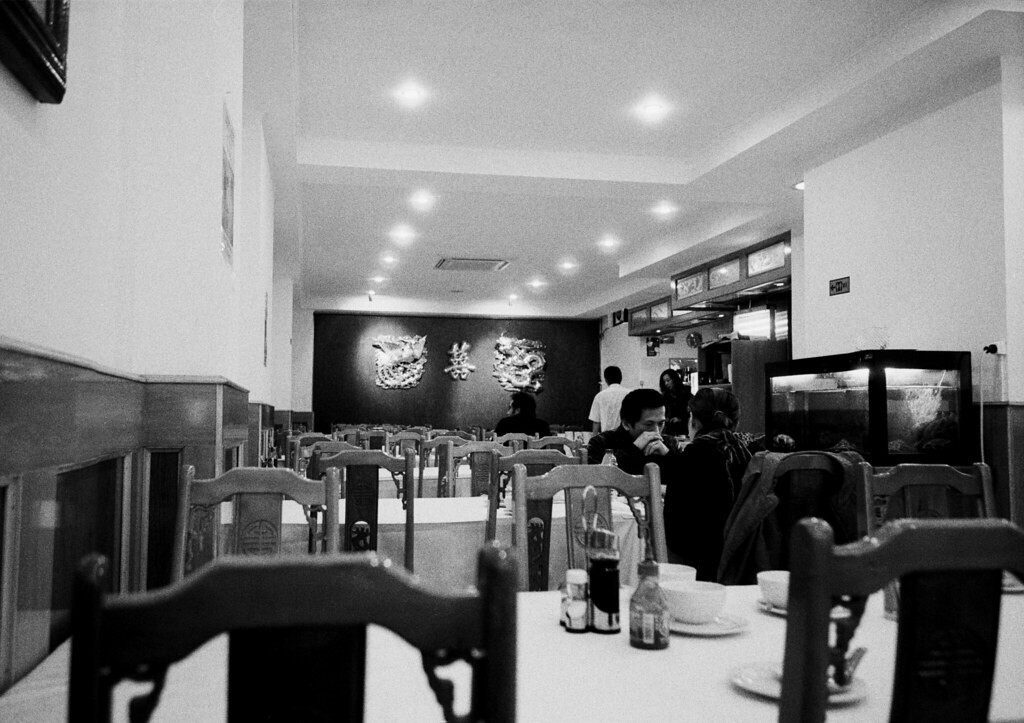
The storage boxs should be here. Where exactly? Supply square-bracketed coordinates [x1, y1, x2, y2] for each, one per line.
[763, 348, 976, 466]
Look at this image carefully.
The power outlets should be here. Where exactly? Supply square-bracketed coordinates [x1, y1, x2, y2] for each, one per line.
[990, 340, 1006, 356]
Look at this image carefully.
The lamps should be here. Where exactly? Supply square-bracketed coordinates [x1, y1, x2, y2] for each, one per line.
[369, 291, 375, 301]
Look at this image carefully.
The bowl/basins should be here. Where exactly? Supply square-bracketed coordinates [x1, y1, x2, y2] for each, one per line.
[658, 564, 696, 584]
[661, 581, 727, 623]
[757, 569, 790, 610]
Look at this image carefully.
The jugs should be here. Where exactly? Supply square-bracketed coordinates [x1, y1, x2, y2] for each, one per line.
[773, 616, 866, 691]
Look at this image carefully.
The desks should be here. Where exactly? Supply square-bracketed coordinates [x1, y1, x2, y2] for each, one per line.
[337, 465, 471, 498]
[0, 580, 1024, 723]
[396, 454, 438, 467]
[218, 493, 645, 592]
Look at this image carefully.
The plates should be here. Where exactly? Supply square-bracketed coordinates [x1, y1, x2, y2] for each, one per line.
[669, 620, 748, 635]
[759, 602, 851, 619]
[732, 672, 871, 702]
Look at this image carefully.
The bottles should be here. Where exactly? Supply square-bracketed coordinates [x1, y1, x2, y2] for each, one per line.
[629, 559, 671, 651]
[590, 528, 621, 634]
[564, 569, 589, 632]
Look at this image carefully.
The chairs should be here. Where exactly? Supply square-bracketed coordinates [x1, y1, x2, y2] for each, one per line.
[65, 424, 1024, 723]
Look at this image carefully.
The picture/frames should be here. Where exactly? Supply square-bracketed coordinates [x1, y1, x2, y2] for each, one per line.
[0, 0, 71, 104]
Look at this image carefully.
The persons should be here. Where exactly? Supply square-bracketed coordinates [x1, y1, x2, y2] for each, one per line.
[663, 387, 794, 584]
[494, 391, 550, 450]
[588, 388, 680, 483]
[588, 366, 633, 436]
[660, 369, 694, 437]
